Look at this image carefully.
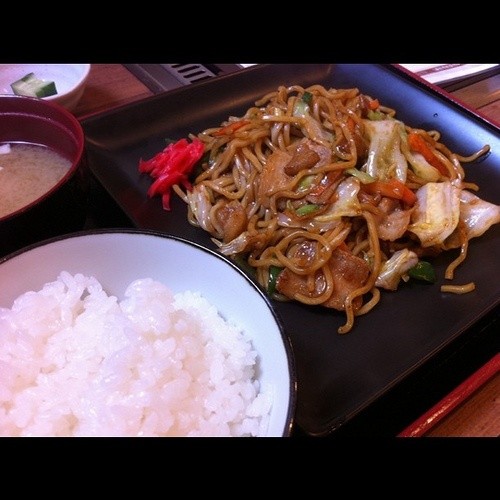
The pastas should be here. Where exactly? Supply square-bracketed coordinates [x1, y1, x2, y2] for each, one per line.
[172, 83, 489, 333]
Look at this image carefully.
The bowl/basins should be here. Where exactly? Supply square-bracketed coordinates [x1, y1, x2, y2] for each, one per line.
[0, 226, 298, 437]
[0, 64, 92, 110]
[1, 94, 89, 253]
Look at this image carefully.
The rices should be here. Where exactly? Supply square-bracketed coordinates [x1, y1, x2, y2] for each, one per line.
[0, 271, 271, 437]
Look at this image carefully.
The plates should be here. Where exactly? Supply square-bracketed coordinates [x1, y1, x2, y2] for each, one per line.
[75, 63, 499, 436]
